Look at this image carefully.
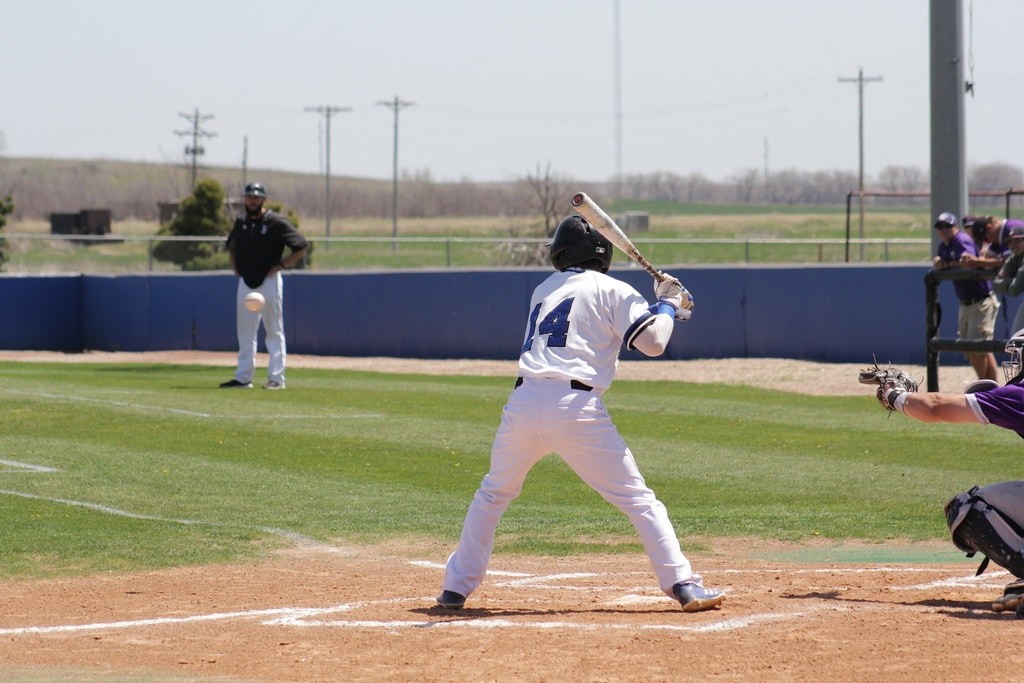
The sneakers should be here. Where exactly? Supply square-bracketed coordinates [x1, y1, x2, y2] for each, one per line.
[437, 588, 466, 608]
[219, 378, 251, 388]
[672, 581, 726, 612]
[263, 380, 287, 390]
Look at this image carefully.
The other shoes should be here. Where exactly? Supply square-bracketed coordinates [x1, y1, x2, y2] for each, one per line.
[992, 589, 1024, 612]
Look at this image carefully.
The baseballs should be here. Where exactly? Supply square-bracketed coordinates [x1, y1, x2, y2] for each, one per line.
[244, 291, 266, 312]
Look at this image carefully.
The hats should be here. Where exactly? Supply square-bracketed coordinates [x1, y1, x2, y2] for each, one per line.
[1000, 220, 1024, 242]
[935, 212, 957, 227]
[963, 216, 976, 227]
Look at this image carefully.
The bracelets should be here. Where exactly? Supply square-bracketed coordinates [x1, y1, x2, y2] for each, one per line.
[281, 262, 285, 269]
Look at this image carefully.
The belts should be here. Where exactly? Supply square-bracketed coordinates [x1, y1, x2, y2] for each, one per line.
[514, 376, 592, 392]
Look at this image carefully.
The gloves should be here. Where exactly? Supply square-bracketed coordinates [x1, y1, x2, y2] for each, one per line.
[652, 270, 683, 311]
[882, 381, 914, 414]
[674, 292, 693, 323]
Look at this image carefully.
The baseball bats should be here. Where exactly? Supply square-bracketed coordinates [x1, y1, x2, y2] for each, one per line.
[571, 191, 666, 284]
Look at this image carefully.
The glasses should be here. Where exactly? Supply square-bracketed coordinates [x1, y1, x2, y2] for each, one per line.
[936, 223, 955, 230]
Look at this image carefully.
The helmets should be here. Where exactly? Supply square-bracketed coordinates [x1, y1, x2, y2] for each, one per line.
[1001, 328, 1024, 354]
[245, 182, 265, 197]
[543, 213, 613, 274]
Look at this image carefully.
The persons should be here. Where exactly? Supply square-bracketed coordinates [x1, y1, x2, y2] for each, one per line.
[960, 216, 1024, 270]
[219, 183, 309, 391]
[962, 215, 977, 237]
[858, 328, 1024, 611]
[933, 213, 1000, 384]
[995, 224, 1024, 337]
[436, 214, 726, 611]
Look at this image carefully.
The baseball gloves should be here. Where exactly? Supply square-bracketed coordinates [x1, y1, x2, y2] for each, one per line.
[859, 365, 919, 413]
